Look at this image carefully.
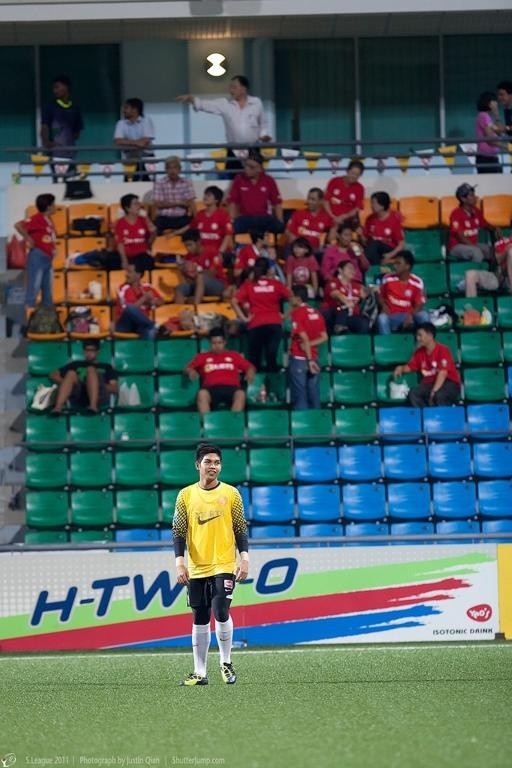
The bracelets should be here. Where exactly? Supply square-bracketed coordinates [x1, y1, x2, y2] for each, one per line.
[496, 118, 500, 122]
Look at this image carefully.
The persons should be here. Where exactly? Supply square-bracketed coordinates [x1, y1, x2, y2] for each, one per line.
[448, 183, 512, 298]
[40, 76, 83, 184]
[48, 338, 119, 415]
[113, 153, 462, 413]
[476, 92, 512, 173]
[497, 82, 512, 144]
[177, 76, 273, 179]
[113, 97, 156, 183]
[14, 194, 57, 308]
[172, 444, 249, 687]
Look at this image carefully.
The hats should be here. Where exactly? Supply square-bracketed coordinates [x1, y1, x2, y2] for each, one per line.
[456, 182, 478, 198]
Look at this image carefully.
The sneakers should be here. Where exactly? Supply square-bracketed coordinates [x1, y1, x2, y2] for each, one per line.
[177, 673, 208, 686]
[220, 662, 236, 684]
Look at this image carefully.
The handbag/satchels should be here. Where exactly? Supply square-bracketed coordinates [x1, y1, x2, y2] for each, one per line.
[23, 306, 64, 334]
[31, 383, 57, 410]
[65, 308, 92, 333]
